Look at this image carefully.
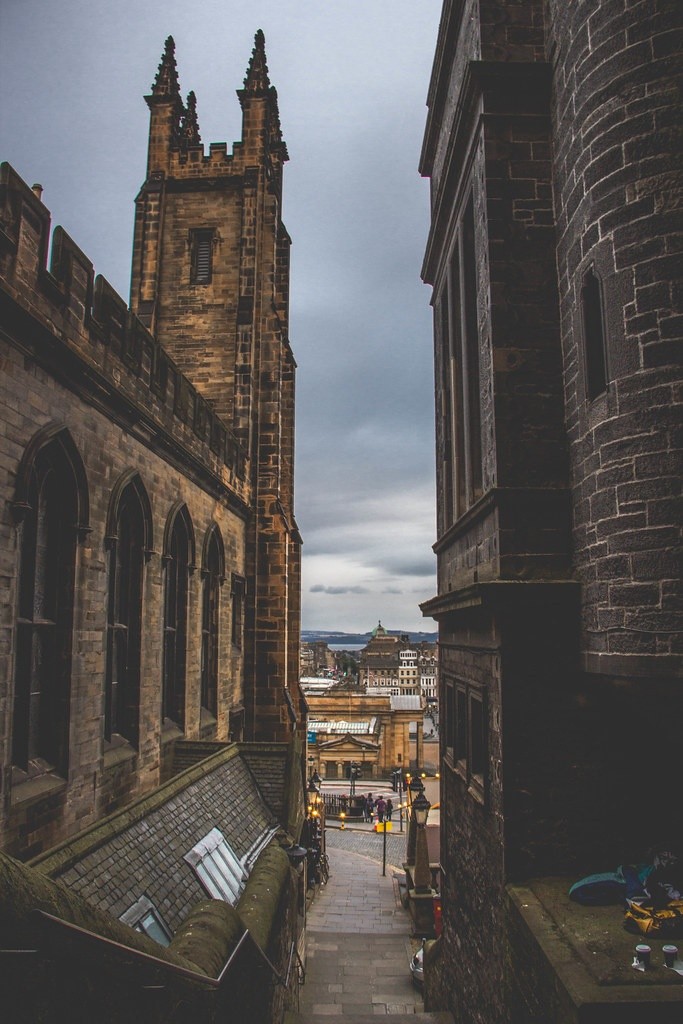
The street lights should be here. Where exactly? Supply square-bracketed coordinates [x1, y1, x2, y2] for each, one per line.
[361, 744, 367, 764]
[411, 786, 433, 893]
[307, 755, 314, 777]
[407, 775, 425, 865]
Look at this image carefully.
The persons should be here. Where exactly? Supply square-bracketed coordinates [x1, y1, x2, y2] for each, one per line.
[387, 799, 393, 821]
[365, 793, 374, 823]
[375, 796, 386, 823]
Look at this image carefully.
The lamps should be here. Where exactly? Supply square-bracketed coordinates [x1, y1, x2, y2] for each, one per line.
[409, 776, 425, 805]
[307, 770, 322, 809]
[412, 788, 431, 827]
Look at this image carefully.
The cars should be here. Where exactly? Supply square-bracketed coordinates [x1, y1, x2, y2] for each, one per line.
[410, 944, 427, 993]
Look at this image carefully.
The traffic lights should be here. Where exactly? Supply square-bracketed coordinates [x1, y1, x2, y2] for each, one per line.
[390, 771, 398, 791]
[402, 775, 407, 791]
[346, 765, 350, 779]
